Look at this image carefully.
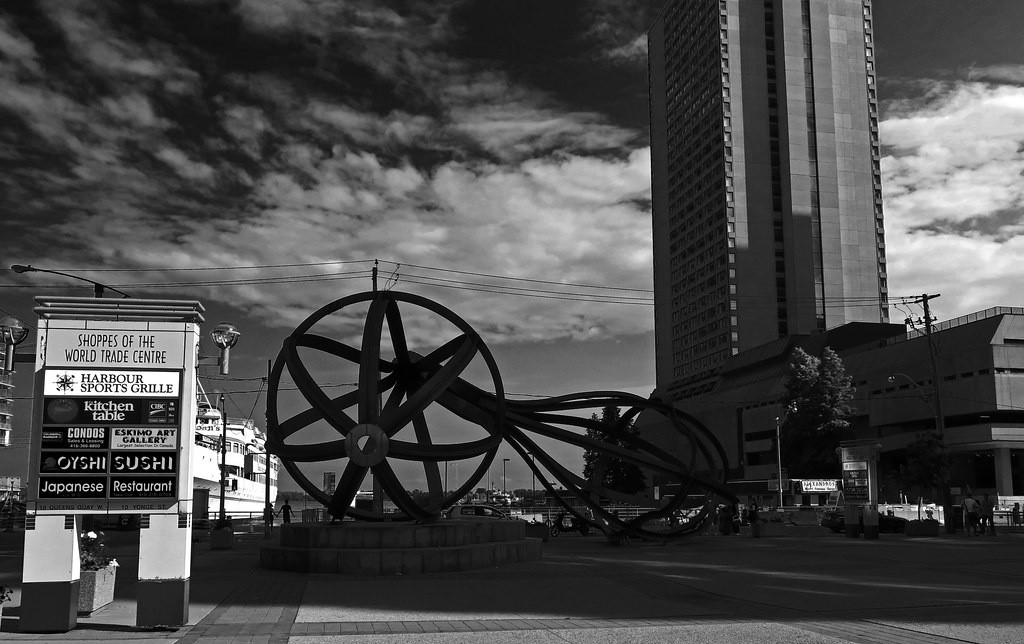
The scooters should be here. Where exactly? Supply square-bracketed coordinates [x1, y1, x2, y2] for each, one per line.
[550, 507, 589, 537]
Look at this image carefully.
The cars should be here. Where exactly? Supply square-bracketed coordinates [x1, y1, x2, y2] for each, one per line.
[817, 508, 908, 534]
[444, 504, 512, 521]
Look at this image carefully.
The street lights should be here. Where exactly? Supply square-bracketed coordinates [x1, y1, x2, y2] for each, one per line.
[775, 401, 797, 507]
[10, 264, 134, 299]
[527, 452, 535, 500]
[212, 392, 234, 528]
[888, 373, 952, 534]
[503, 458, 510, 489]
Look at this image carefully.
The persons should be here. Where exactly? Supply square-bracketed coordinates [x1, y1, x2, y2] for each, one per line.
[277, 499, 295, 523]
[216, 513, 231, 530]
[975, 493, 996, 536]
[747, 499, 759, 538]
[731, 501, 740, 536]
[608, 510, 631, 545]
[965, 493, 984, 537]
[703, 505, 721, 536]
[531, 518, 537, 523]
[263, 503, 277, 533]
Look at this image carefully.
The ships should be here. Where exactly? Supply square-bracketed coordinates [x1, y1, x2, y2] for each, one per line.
[194, 371, 279, 522]
